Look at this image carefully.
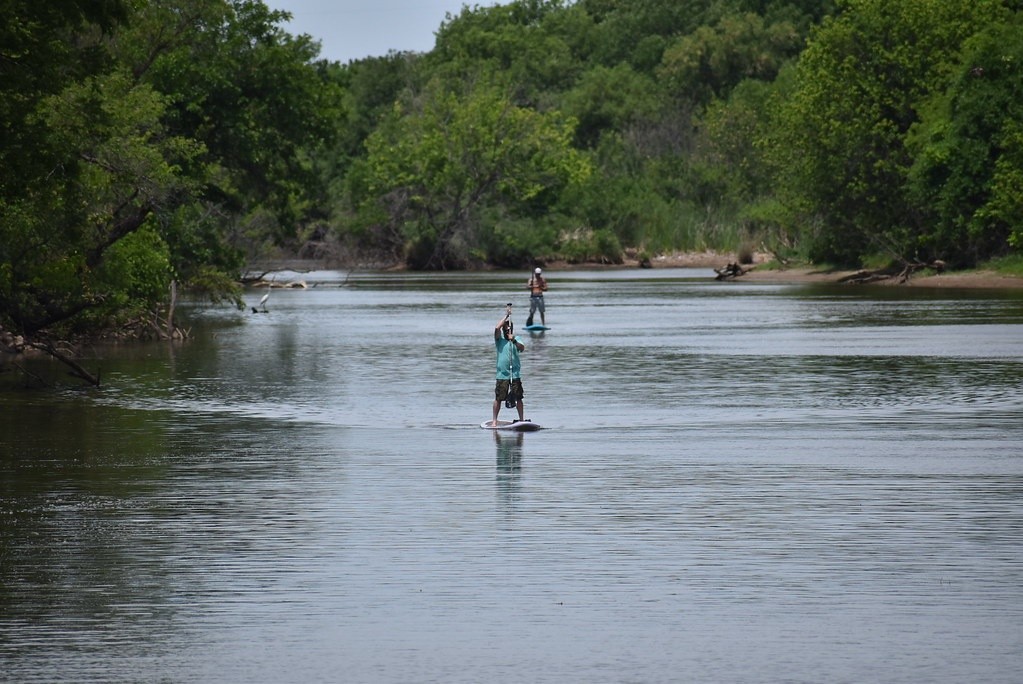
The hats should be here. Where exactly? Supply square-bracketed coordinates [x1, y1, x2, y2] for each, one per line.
[535, 268, 542, 274]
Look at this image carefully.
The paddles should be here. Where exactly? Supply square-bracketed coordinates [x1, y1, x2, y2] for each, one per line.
[526, 259, 533, 327]
[505, 302, 516, 408]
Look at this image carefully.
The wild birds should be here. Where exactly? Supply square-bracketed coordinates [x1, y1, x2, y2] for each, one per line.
[259, 284, 277, 313]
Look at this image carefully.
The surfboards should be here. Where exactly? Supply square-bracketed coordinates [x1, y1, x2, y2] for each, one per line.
[481, 419, 541, 430]
[522, 324, 551, 330]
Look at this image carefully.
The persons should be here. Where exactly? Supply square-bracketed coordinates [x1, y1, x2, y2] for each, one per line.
[733, 264, 737, 272]
[527, 267, 548, 326]
[491, 306, 525, 427]
